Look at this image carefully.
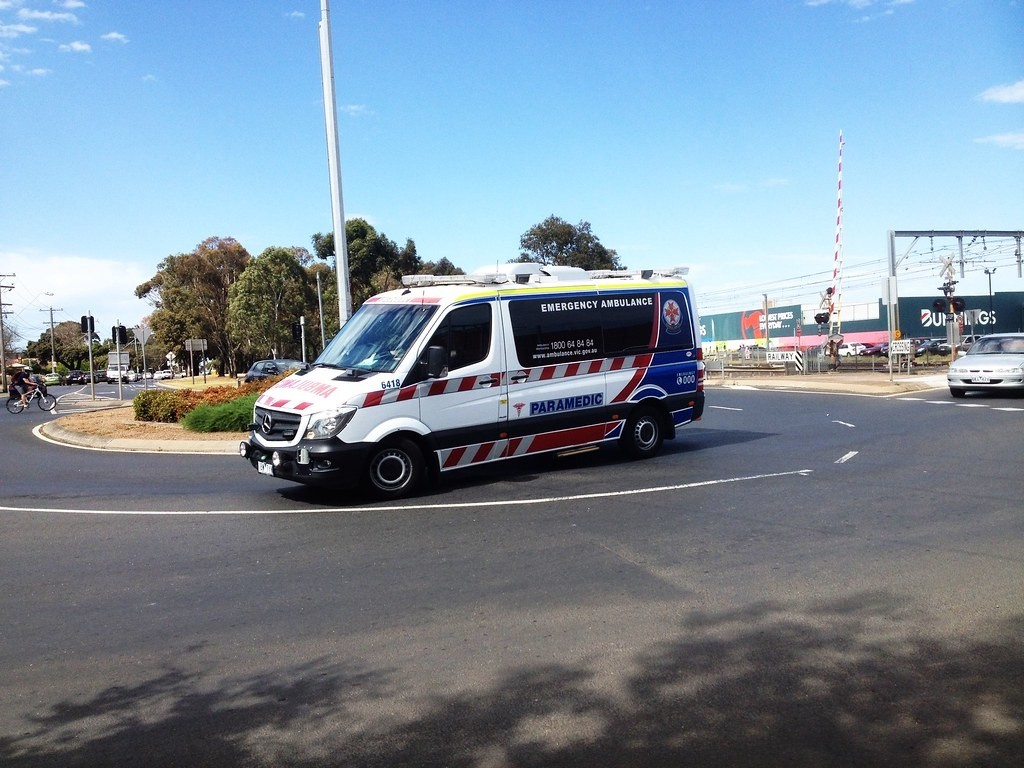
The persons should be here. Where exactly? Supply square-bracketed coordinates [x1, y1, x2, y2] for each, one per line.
[11, 367, 38, 408]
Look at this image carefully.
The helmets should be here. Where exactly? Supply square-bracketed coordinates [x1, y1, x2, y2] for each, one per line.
[23, 365, 35, 370]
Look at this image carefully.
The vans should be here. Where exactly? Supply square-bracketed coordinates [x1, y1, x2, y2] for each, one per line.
[106, 364, 129, 384]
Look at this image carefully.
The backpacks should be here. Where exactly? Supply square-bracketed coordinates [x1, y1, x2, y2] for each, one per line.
[11, 371, 23, 382]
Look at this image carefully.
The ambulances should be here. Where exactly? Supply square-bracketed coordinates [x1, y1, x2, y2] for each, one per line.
[238, 260, 713, 510]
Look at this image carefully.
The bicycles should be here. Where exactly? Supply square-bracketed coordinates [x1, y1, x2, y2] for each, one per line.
[5, 382, 57, 416]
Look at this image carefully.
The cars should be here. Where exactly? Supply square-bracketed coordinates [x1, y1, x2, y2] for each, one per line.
[96, 370, 106, 382]
[820, 334, 985, 357]
[127, 370, 175, 382]
[947, 332, 1024, 397]
[46, 372, 61, 386]
[85, 371, 95, 382]
[8, 373, 47, 398]
[64, 370, 86, 386]
[245, 358, 303, 384]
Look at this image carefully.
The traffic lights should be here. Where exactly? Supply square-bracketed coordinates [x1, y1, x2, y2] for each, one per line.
[933, 298, 946, 312]
[952, 298, 965, 312]
[815, 314, 829, 324]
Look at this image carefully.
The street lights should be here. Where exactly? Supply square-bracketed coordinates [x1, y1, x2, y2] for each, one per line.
[761, 293, 771, 351]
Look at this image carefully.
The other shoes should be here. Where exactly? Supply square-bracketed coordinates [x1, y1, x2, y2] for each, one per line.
[23, 404, 28, 408]
